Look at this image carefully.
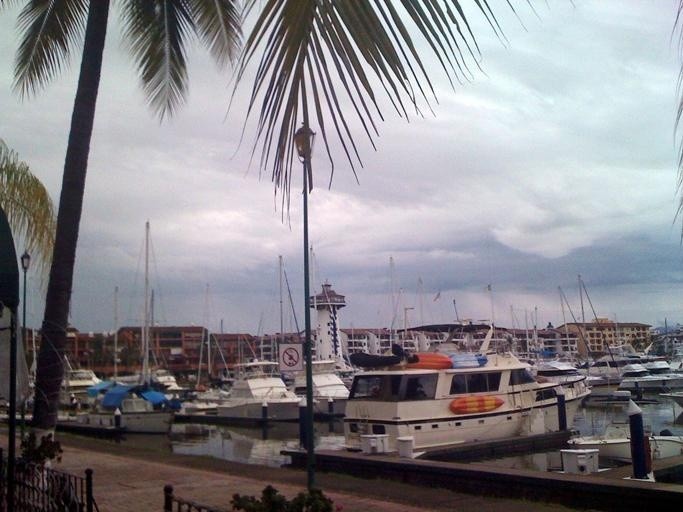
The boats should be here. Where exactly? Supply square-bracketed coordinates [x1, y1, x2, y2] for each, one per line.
[340, 343, 592, 459]
[565, 420, 683, 471]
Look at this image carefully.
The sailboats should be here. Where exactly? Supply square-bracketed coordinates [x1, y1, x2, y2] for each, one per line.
[510, 271, 683, 405]
[56, 219, 352, 435]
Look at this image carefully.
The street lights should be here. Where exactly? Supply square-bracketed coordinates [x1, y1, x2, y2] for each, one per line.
[19, 249, 30, 444]
[290, 118, 320, 498]
